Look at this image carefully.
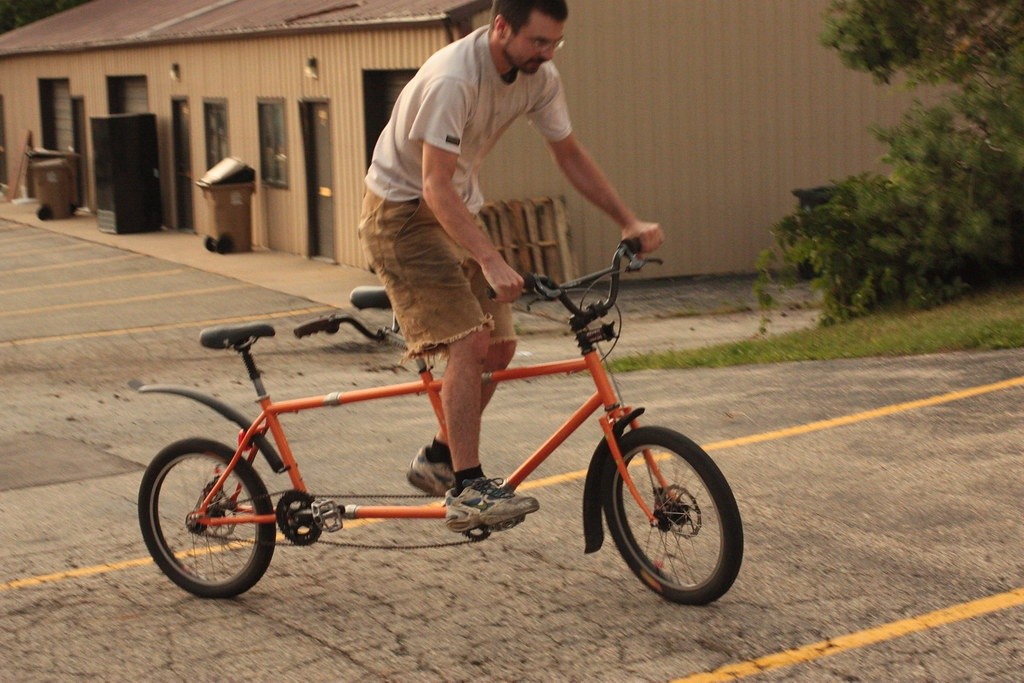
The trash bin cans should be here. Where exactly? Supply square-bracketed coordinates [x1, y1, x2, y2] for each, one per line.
[791, 187, 835, 279]
[194, 156, 257, 255]
[29, 146, 81, 221]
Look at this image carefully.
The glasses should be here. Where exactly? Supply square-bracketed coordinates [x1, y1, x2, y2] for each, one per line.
[506, 23, 564, 53]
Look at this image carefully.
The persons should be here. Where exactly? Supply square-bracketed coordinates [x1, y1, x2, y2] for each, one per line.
[362, 0, 666, 530]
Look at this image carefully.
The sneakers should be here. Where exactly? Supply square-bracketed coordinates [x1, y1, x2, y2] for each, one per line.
[406, 446, 459, 497]
[444, 476, 539, 533]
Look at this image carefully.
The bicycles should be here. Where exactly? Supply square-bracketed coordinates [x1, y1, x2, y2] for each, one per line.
[136, 236, 744, 607]
